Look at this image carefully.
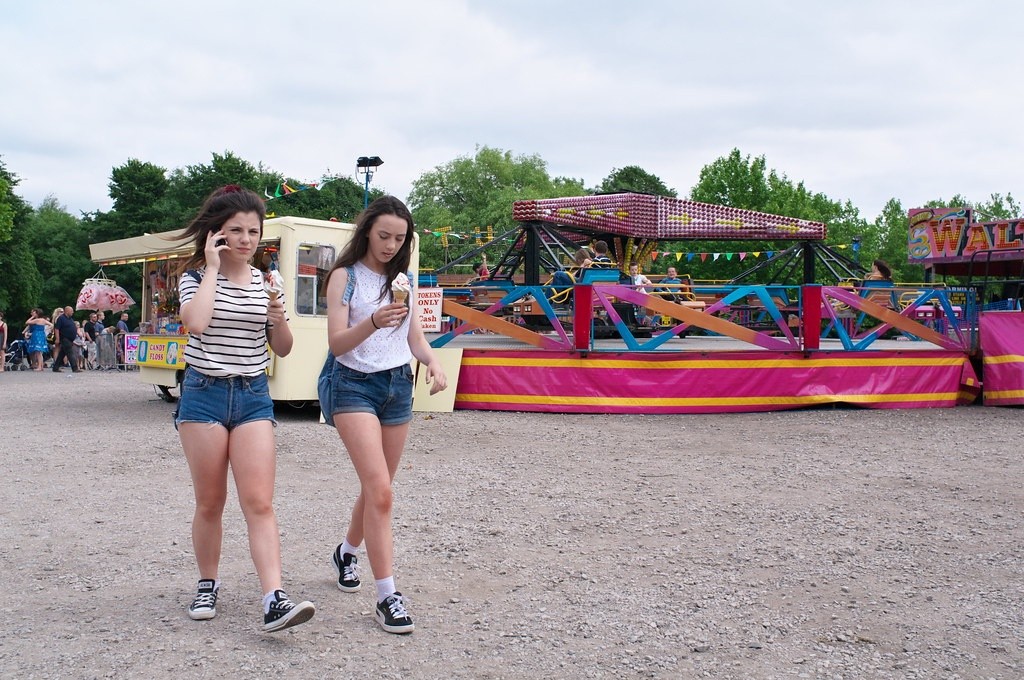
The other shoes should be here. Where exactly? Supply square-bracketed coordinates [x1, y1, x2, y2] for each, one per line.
[52, 368, 62, 372]
[72, 369, 83, 372]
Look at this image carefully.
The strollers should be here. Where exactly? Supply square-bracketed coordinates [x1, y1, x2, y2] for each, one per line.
[5, 336, 27, 371]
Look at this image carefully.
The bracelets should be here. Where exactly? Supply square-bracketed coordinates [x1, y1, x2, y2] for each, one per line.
[372, 313, 380, 330]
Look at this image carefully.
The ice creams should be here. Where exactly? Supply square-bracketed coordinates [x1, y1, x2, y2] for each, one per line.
[263, 269, 284, 301]
[391, 272, 410, 320]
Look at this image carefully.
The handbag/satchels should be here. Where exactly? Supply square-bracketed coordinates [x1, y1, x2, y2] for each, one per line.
[46, 334, 56, 345]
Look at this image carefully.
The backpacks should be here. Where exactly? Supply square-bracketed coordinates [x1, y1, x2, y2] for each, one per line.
[318, 265, 414, 424]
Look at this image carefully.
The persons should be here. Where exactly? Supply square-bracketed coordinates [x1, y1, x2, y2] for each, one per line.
[320, 194, 449, 633]
[864, 260, 890, 280]
[0, 306, 130, 374]
[471, 241, 682, 323]
[154, 184, 315, 633]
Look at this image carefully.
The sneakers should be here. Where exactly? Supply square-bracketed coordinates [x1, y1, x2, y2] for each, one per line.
[375, 592, 415, 632]
[189, 579, 222, 619]
[264, 590, 316, 633]
[330, 543, 361, 594]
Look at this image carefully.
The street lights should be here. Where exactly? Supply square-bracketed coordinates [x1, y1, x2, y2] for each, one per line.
[355, 156, 384, 211]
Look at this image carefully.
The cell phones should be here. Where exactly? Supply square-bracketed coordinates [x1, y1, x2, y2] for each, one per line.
[212, 228, 229, 252]
